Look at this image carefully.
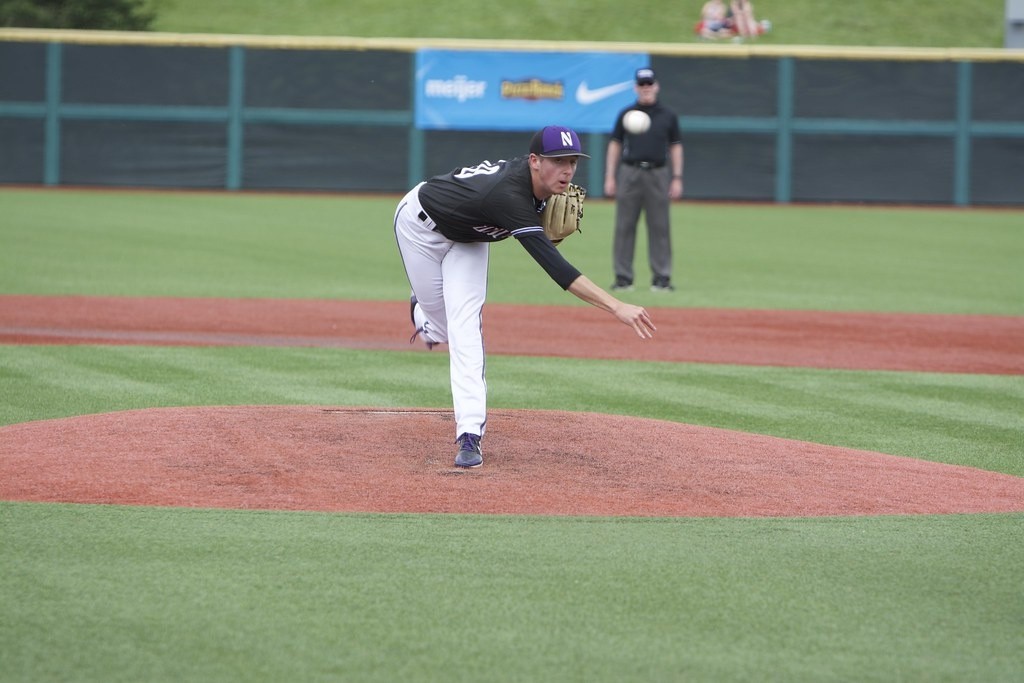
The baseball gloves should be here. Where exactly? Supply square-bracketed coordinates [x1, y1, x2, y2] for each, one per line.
[542, 181, 588, 247]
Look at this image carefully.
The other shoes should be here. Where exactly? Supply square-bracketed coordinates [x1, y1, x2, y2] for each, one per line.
[611, 279, 632, 290]
[651, 275, 672, 291]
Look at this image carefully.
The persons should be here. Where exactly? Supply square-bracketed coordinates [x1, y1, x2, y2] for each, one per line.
[699, 0, 760, 38]
[393, 125, 656, 468]
[604, 67, 684, 293]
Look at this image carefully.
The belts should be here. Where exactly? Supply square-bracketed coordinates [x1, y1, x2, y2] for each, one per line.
[623, 159, 663, 169]
[418, 211, 440, 233]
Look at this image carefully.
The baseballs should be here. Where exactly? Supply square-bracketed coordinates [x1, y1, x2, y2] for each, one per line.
[622, 109, 651, 134]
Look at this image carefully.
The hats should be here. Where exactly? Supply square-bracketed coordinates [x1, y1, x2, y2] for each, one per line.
[529, 125, 591, 161]
[635, 68, 655, 83]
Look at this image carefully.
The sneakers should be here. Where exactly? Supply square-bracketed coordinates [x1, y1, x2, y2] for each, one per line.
[409, 291, 438, 346]
[454, 432, 483, 468]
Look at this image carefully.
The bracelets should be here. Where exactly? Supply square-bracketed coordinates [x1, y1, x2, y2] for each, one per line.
[671, 175, 683, 181]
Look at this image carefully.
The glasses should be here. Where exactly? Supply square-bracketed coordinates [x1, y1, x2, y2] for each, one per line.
[637, 81, 653, 86]
